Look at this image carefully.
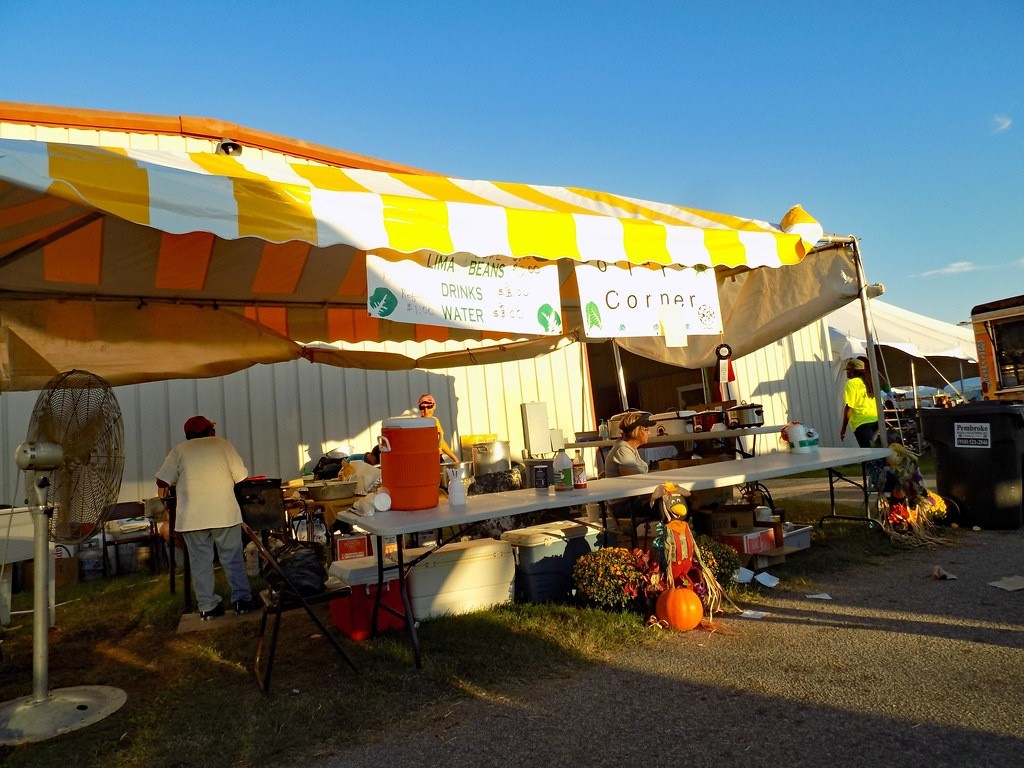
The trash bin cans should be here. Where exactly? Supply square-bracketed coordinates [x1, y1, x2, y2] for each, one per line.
[904, 401, 1024, 530]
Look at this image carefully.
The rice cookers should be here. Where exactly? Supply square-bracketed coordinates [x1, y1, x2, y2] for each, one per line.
[726, 401, 765, 430]
[693, 407, 724, 433]
[649, 408, 697, 436]
[607, 408, 652, 438]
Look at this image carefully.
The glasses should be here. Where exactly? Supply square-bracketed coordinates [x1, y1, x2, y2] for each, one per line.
[419, 405, 434, 410]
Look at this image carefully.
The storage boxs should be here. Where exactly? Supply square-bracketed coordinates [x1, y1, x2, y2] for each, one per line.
[658, 456, 813, 569]
[328, 518, 617, 642]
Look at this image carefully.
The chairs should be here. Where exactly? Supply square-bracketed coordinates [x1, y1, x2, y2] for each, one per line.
[100, 501, 169, 582]
[242, 523, 362, 695]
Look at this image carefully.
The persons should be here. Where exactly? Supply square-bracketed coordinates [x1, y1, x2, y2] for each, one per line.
[840, 358, 884, 495]
[604, 413, 662, 519]
[885, 400, 910, 429]
[155, 416, 264, 621]
[935, 397, 945, 408]
[418, 393, 459, 463]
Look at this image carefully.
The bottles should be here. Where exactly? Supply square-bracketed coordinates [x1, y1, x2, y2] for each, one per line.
[573, 450, 587, 489]
[787, 421, 818, 454]
[448, 480, 466, 505]
[553, 449, 575, 492]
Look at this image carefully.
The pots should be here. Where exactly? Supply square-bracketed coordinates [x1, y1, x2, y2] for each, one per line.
[307, 482, 358, 499]
[439, 461, 474, 488]
[472, 441, 513, 476]
[526, 459, 572, 485]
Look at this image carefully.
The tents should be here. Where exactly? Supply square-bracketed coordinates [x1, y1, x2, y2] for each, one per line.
[0, 99, 894, 529]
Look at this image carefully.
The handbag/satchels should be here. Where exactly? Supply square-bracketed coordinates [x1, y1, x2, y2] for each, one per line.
[262, 538, 329, 597]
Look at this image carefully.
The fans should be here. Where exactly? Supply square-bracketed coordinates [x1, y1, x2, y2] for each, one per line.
[0, 369, 126, 746]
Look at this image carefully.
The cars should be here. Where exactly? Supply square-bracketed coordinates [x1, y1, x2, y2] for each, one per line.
[880, 385, 948, 432]
[943, 377, 983, 402]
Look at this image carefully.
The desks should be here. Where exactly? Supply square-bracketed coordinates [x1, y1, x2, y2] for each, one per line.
[618, 448, 895, 557]
[338, 478, 722, 670]
[565, 425, 786, 523]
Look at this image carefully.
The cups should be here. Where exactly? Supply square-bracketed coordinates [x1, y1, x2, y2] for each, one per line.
[586, 503, 599, 521]
[535, 466, 548, 489]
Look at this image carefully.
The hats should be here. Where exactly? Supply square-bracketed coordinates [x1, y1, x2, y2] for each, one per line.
[418, 394, 435, 406]
[183, 416, 216, 438]
[618, 414, 656, 432]
[842, 359, 864, 370]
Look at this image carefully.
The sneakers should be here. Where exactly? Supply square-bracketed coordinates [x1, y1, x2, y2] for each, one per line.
[232, 603, 249, 615]
[200, 605, 225, 621]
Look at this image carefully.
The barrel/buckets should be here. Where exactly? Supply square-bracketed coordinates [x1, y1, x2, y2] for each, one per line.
[377, 417, 441, 510]
